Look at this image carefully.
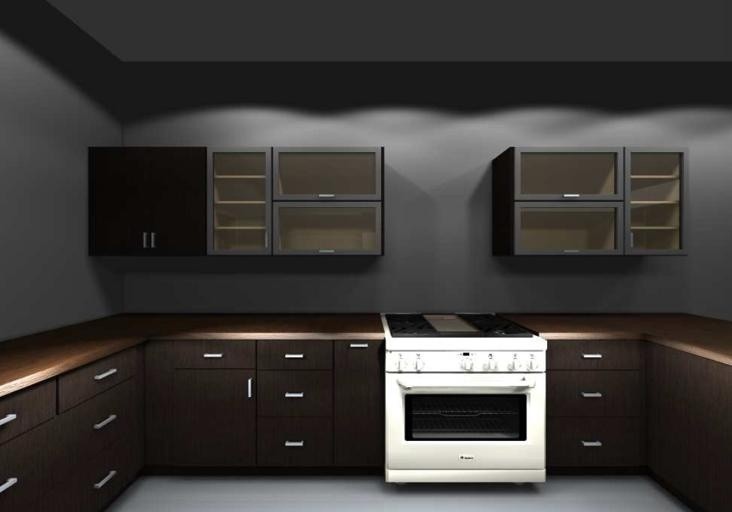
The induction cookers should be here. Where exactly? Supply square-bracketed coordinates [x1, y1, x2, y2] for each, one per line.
[380, 309, 538, 338]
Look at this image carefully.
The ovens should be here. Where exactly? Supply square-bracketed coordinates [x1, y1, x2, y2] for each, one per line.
[383, 339, 548, 486]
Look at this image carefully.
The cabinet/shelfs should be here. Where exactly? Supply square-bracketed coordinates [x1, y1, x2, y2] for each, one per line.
[256, 330, 334, 474]
[491, 146, 624, 201]
[146, 330, 256, 475]
[334, 333, 385, 474]
[620, 147, 687, 257]
[546, 329, 644, 476]
[270, 146, 386, 201]
[58, 331, 146, 512]
[86, 146, 208, 259]
[491, 201, 624, 258]
[0, 364, 58, 512]
[204, 146, 272, 258]
[271, 202, 384, 255]
